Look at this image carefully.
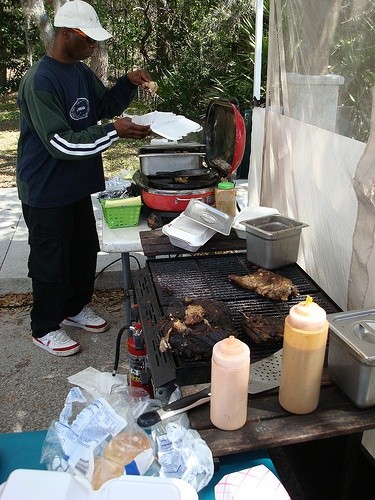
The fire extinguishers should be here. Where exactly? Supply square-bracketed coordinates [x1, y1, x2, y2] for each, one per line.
[112, 322, 153, 397]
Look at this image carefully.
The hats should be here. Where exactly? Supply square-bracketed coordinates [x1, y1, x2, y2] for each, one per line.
[54, 0, 113, 42]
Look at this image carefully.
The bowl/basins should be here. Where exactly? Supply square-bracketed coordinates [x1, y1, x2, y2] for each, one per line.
[162, 212, 219, 254]
[229, 206, 280, 239]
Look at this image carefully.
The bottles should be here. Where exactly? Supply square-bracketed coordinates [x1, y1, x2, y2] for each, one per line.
[215, 182, 236, 218]
[279, 294, 329, 414]
[210, 335, 250, 431]
[90, 432, 149, 488]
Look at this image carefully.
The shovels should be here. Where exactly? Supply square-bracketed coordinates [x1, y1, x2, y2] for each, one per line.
[137, 348, 283, 427]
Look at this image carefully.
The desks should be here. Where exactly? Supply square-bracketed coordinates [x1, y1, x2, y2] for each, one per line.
[102, 188, 159, 342]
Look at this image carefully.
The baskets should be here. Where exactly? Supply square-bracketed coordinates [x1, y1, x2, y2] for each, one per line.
[99, 200, 143, 229]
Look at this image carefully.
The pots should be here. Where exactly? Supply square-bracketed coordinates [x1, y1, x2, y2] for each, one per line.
[132, 97, 246, 211]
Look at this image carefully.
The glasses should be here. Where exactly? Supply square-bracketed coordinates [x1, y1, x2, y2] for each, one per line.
[72, 28, 95, 43]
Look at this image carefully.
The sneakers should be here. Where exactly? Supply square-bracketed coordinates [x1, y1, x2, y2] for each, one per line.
[62, 304, 109, 332]
[33, 328, 81, 356]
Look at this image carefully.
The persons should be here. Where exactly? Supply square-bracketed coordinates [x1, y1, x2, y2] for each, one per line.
[16, 0, 153, 357]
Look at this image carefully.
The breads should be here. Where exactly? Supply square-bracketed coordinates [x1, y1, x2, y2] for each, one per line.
[90, 455, 124, 490]
[103, 431, 150, 467]
